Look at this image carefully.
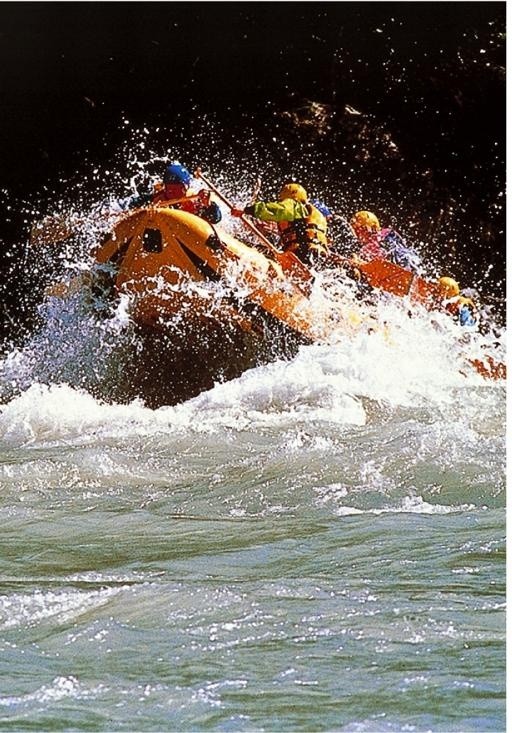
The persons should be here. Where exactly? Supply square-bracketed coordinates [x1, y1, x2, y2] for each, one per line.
[45, 162, 501, 354]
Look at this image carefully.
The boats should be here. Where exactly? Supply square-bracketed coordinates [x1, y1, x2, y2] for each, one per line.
[71, 207, 506, 396]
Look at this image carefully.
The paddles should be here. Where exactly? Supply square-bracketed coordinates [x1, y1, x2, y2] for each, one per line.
[29, 195, 202, 247]
[194, 168, 317, 292]
[408, 277, 438, 311]
[43, 276, 88, 301]
[333, 251, 413, 298]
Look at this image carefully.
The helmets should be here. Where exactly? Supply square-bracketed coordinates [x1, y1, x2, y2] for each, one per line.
[163, 165, 191, 183]
[312, 197, 331, 216]
[438, 276, 460, 300]
[349, 210, 382, 234]
[279, 183, 308, 203]
